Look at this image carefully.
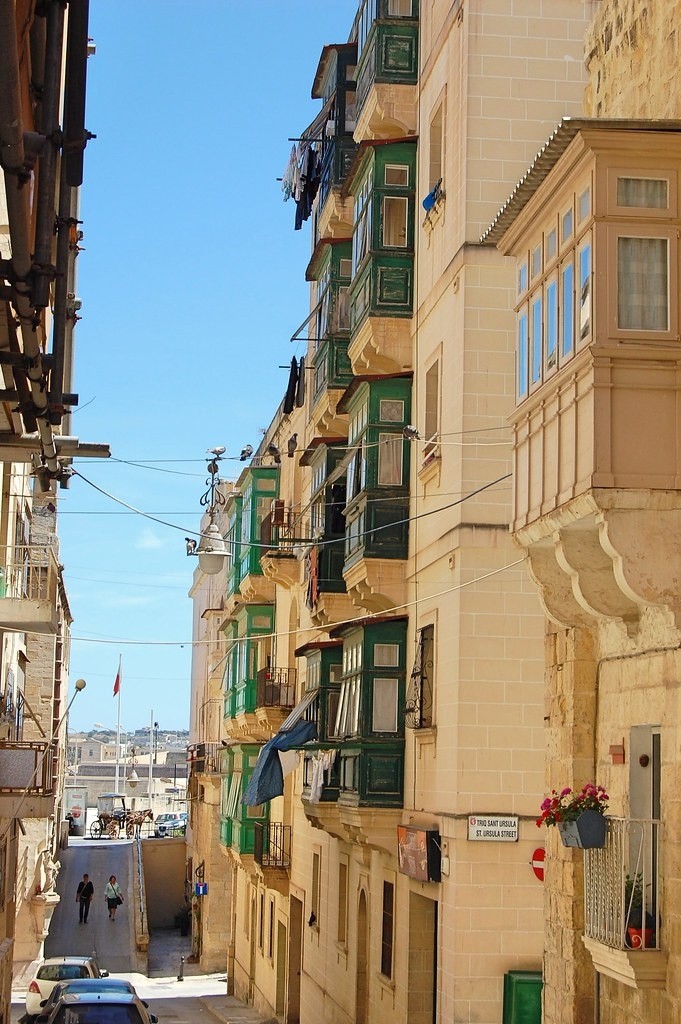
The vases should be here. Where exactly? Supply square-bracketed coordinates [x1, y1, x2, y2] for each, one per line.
[557, 810, 606, 850]
[266, 680, 271, 682]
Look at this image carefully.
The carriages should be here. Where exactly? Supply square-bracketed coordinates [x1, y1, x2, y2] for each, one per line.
[90, 792, 155, 840]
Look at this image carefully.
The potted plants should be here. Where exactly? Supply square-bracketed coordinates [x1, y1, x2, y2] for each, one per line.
[322, 768, 340, 802]
[617, 871, 655, 949]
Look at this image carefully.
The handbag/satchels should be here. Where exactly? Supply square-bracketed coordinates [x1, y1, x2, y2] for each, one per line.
[117, 896, 123, 905]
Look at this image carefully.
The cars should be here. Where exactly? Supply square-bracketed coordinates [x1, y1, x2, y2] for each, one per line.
[154, 814, 188, 837]
[25, 956, 110, 1019]
[35, 978, 159, 1024]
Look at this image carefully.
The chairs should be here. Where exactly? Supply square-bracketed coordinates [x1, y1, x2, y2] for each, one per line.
[111, 807, 125, 820]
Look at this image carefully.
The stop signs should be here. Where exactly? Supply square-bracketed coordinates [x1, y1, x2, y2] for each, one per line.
[532, 848, 545, 881]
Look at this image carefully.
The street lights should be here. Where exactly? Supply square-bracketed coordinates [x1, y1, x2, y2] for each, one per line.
[116, 723, 128, 792]
[93, 723, 118, 791]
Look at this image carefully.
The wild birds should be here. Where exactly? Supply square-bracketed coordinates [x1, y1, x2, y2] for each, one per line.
[288, 433, 298, 457]
[205, 446, 226, 457]
[267, 442, 281, 463]
[240, 445, 253, 461]
[185, 538, 196, 553]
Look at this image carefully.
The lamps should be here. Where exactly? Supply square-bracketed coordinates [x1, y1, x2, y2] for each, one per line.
[194, 460, 232, 574]
[127, 746, 141, 788]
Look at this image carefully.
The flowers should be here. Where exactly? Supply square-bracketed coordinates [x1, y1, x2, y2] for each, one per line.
[532, 782, 609, 826]
[266, 674, 271, 679]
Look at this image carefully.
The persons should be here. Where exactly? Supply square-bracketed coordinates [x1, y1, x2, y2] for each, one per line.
[104, 875, 124, 921]
[76, 874, 94, 924]
[65, 813, 74, 835]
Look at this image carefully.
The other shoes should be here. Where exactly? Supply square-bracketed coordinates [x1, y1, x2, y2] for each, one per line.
[109, 914, 111, 918]
[111, 918, 114, 921]
[85, 922, 88, 924]
[79, 921, 83, 924]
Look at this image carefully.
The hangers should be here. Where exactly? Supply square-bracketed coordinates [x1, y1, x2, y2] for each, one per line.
[291, 138, 317, 156]
